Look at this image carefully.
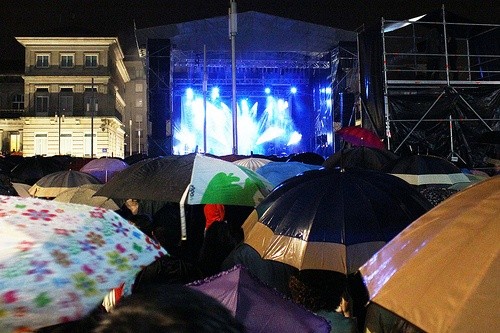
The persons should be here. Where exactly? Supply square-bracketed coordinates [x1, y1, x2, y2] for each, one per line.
[90, 284, 248, 333]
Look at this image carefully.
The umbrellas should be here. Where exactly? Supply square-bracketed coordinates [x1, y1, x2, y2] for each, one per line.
[0, 125, 500, 333]
